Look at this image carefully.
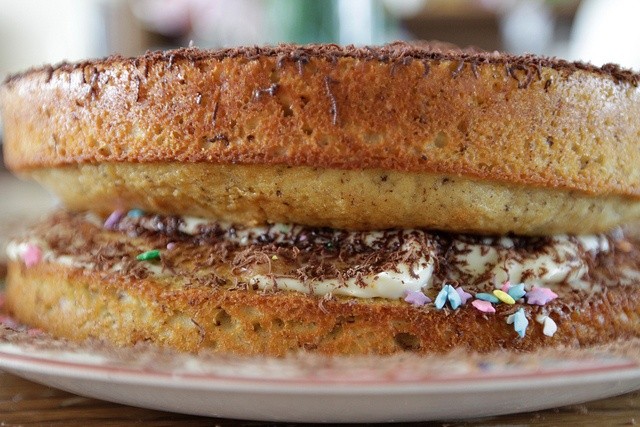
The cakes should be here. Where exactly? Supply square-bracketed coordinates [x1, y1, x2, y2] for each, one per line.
[2, 40, 640, 376]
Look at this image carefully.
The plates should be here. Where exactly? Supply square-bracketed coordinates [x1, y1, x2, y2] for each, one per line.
[1, 339, 640, 420]
